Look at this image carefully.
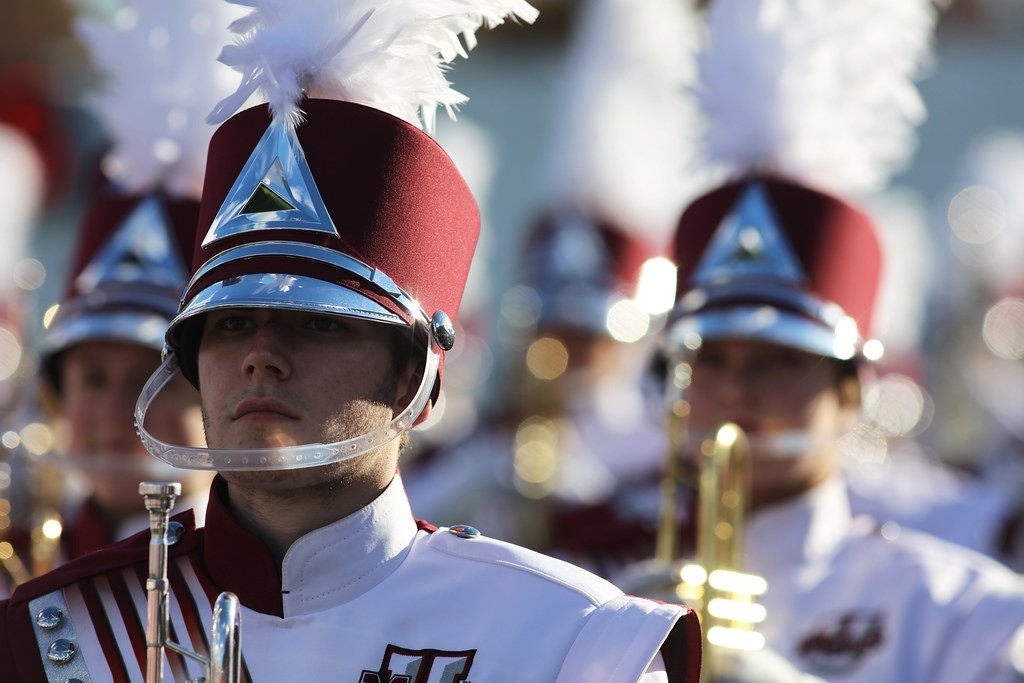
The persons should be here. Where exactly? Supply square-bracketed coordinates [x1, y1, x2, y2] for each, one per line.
[0, 0, 701, 683]
[1, 0, 1023, 683]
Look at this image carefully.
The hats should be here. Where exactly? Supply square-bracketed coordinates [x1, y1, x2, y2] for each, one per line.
[662, 173, 882, 363]
[165, 100, 480, 410]
[36, 185, 204, 402]
[520, 214, 670, 341]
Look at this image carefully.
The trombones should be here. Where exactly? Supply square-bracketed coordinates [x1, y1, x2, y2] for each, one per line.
[658, 398, 749, 683]
[138, 480, 243, 683]
